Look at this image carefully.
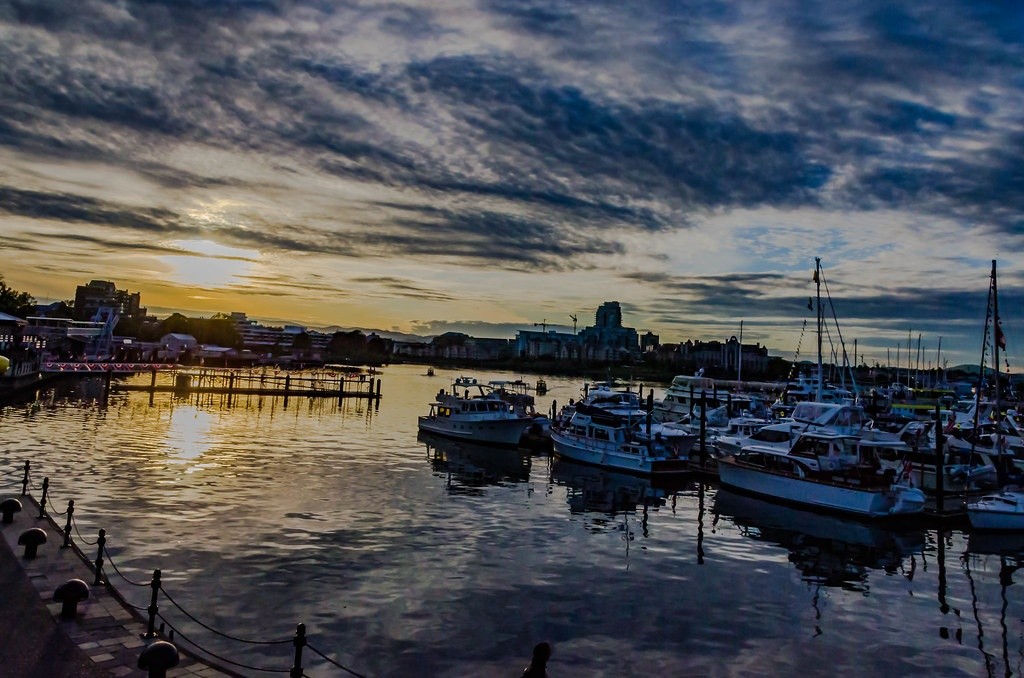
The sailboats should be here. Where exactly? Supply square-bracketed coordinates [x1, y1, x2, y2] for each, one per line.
[964, 260, 1024, 529]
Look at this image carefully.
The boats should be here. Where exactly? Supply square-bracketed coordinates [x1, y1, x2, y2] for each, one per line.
[418, 378, 533, 444]
[644, 256, 1024, 511]
[472, 380, 552, 450]
[417, 429, 532, 497]
[706, 402, 926, 515]
[715, 488, 928, 593]
[551, 374, 701, 479]
[550, 455, 699, 569]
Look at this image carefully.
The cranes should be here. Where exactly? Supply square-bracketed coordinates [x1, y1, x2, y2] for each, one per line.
[569, 314, 577, 335]
[534, 318, 562, 333]
